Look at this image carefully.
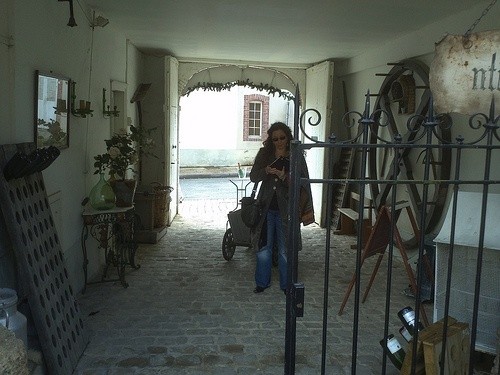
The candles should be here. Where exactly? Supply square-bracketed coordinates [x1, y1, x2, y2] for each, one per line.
[80, 100, 86, 109]
[87, 102, 90, 110]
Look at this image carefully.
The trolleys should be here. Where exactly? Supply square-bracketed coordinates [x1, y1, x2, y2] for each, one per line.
[222, 180, 278, 267]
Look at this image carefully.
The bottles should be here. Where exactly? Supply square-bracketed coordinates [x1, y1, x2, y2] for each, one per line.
[398, 306, 424, 336]
[399, 326, 413, 343]
[4, 145, 61, 177]
[379, 334, 405, 371]
[0, 288, 27, 348]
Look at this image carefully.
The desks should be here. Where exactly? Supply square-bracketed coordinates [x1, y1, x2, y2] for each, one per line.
[80, 205, 141, 295]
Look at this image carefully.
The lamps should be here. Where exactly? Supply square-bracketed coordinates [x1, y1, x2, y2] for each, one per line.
[58, 0, 78, 27]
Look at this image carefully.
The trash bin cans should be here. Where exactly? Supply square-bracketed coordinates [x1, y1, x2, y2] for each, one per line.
[134, 185, 174, 230]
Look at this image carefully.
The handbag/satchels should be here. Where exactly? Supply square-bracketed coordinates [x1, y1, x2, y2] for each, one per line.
[239, 191, 264, 229]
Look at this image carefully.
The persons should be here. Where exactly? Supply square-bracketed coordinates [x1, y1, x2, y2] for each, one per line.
[250, 122, 293, 296]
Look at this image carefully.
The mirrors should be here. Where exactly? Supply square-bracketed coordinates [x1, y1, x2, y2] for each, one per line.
[34, 70, 71, 152]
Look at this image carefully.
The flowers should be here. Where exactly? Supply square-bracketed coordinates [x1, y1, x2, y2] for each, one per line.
[93, 125, 156, 175]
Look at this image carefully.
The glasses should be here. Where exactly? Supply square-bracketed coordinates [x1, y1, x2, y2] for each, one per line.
[272, 135, 286, 142]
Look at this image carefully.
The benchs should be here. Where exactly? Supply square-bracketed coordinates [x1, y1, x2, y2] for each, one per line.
[333, 191, 373, 250]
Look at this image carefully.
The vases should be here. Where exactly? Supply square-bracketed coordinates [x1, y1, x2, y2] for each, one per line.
[89, 173, 117, 210]
[110, 165, 137, 207]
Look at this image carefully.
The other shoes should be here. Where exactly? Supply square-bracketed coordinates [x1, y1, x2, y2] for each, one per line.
[253, 288, 264, 293]
[282, 288, 286, 295]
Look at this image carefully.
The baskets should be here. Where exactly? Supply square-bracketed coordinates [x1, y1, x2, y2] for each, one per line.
[137, 182, 169, 209]
